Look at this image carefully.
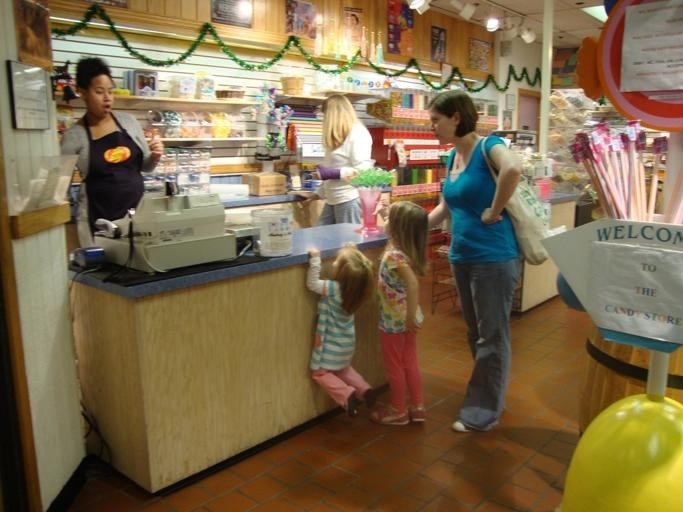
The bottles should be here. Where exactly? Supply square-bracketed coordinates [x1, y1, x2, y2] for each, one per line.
[403, 163, 445, 185]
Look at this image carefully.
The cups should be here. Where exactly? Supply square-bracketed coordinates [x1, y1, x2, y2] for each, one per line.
[355, 188, 383, 235]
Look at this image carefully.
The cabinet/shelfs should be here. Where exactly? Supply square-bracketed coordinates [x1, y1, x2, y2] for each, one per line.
[429, 240, 458, 314]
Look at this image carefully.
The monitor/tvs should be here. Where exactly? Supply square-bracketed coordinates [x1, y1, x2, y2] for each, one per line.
[136, 195, 186, 215]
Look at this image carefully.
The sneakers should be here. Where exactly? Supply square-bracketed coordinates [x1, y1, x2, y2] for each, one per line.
[452, 420, 479, 432]
[347, 391, 359, 417]
[369, 404, 427, 426]
[365, 388, 376, 409]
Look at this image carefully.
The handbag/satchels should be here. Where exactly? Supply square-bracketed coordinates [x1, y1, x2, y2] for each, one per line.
[481, 133, 549, 264]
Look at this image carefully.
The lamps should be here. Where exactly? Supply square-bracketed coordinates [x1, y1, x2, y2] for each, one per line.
[407, 0, 537, 44]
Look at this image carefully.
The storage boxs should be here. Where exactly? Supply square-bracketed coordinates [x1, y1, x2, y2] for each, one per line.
[248, 172, 286, 195]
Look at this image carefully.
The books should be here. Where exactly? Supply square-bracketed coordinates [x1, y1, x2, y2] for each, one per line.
[122, 69, 159, 98]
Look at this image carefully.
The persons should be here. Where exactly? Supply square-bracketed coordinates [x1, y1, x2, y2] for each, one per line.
[349, 13, 363, 44]
[294, 95, 375, 226]
[57, 55, 164, 244]
[369, 200, 435, 428]
[433, 30, 447, 62]
[305, 238, 381, 421]
[420, 89, 531, 436]
